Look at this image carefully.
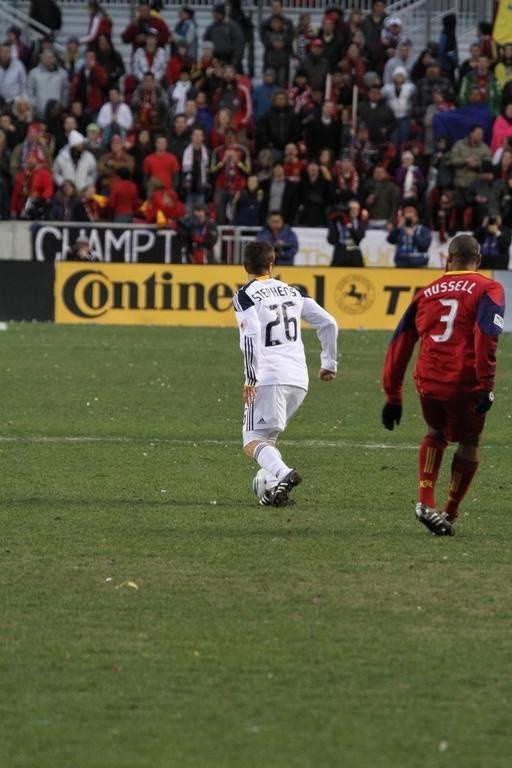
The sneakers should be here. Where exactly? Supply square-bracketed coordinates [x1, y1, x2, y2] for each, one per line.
[259, 469, 301, 507]
[416, 502, 457, 536]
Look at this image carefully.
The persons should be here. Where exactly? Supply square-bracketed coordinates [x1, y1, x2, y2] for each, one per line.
[3, 0, 512, 270]
[232, 241, 339, 505]
[383, 234, 504, 534]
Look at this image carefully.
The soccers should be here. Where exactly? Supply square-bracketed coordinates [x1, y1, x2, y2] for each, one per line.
[252, 467, 281, 500]
